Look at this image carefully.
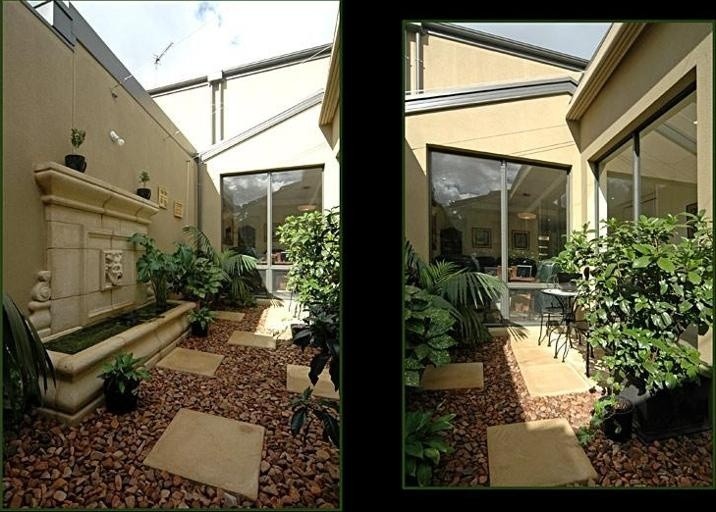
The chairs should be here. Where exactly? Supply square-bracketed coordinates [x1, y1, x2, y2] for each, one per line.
[535, 269, 599, 378]
[495, 264, 518, 282]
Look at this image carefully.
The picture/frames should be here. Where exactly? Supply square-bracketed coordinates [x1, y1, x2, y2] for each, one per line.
[472, 227, 493, 249]
[511, 229, 531, 251]
[685, 202, 699, 239]
[264, 222, 281, 242]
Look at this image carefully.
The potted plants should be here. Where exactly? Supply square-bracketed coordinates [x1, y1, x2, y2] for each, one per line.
[575, 376, 635, 448]
[183, 301, 217, 339]
[94, 347, 154, 415]
[135, 169, 152, 200]
[63, 126, 88, 174]
